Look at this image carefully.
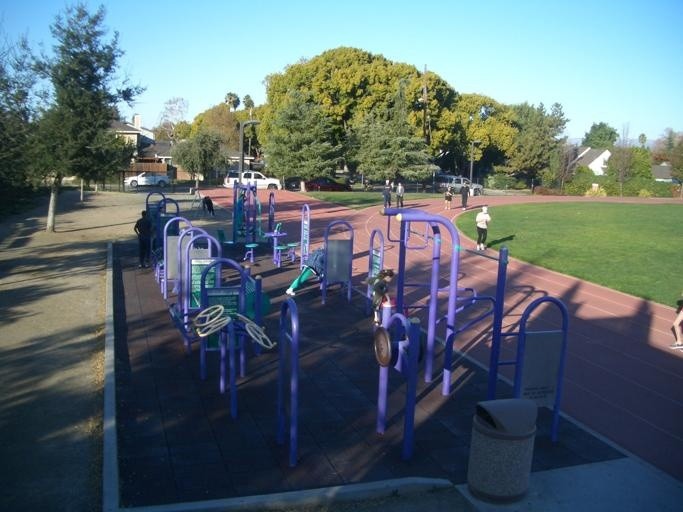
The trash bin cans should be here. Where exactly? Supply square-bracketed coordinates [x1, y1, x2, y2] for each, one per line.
[190, 187, 195, 195]
[468, 399, 538, 505]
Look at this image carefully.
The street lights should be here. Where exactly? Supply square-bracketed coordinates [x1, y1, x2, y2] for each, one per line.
[468, 138, 481, 197]
[237, 119, 261, 188]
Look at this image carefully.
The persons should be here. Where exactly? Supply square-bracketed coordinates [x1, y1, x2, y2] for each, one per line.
[371, 270, 395, 325]
[205, 196, 215, 217]
[396, 181, 404, 209]
[134, 211, 157, 268]
[669, 299, 683, 349]
[444, 184, 452, 210]
[383, 179, 392, 209]
[476, 206, 491, 251]
[460, 182, 469, 211]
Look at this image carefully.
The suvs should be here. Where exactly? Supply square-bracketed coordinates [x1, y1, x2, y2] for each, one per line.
[435, 174, 484, 196]
[222, 170, 282, 191]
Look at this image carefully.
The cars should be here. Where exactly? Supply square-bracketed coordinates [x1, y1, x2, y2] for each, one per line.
[304, 176, 352, 192]
[282, 176, 308, 191]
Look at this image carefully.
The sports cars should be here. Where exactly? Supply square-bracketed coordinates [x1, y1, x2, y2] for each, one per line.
[123, 171, 170, 187]
